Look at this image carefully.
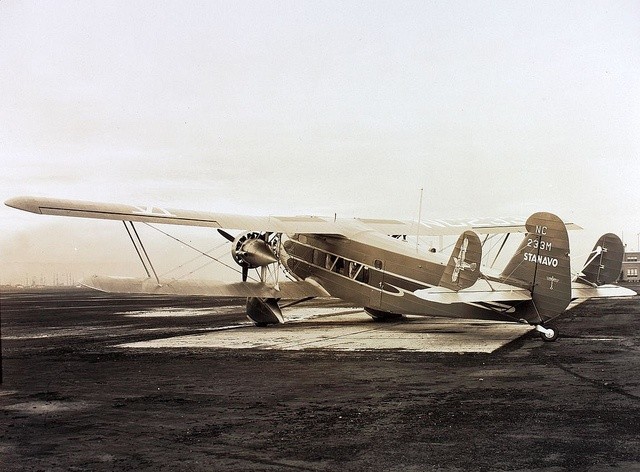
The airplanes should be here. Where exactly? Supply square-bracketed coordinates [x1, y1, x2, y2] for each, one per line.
[4, 196, 640, 342]
